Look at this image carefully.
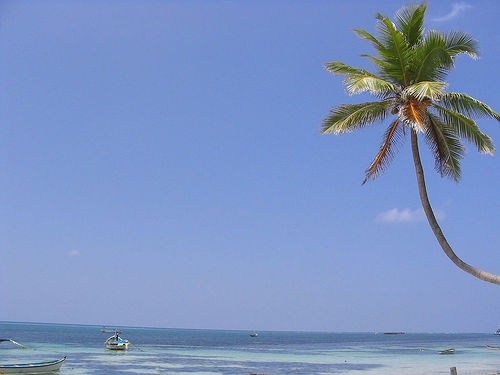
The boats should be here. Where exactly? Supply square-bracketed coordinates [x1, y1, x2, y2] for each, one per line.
[105, 329, 130, 350]
[249, 332, 259, 337]
[0, 337, 67, 373]
[494, 328, 500, 335]
